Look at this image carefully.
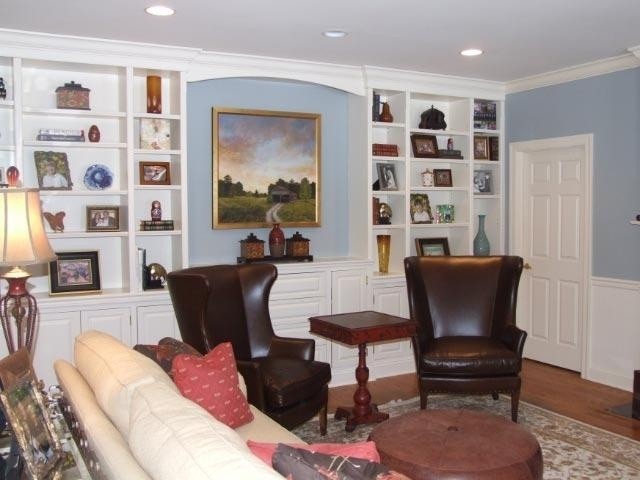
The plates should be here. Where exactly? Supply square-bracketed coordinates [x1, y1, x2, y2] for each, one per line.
[84, 164, 112, 190]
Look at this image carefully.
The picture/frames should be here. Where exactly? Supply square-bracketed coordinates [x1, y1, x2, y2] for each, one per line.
[411, 236, 453, 258]
[137, 161, 171, 187]
[410, 192, 434, 225]
[473, 169, 495, 196]
[47, 249, 103, 297]
[376, 162, 399, 192]
[1, 370, 65, 479]
[83, 203, 122, 232]
[409, 134, 441, 159]
[431, 169, 454, 189]
[208, 103, 324, 233]
[472, 134, 489, 162]
[31, 149, 75, 190]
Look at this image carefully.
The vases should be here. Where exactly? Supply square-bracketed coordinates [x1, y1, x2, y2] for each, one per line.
[144, 74, 163, 115]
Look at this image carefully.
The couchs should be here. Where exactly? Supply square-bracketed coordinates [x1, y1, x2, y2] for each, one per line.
[51, 330, 350, 480]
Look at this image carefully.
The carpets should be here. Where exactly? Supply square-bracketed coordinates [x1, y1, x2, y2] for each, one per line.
[293, 374, 640, 478]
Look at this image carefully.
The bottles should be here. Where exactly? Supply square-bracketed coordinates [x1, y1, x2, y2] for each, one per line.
[420, 167, 434, 186]
[448, 138, 453, 150]
[8, 166, 20, 186]
[150, 200, 162, 221]
[88, 124, 101, 142]
[380, 104, 393, 122]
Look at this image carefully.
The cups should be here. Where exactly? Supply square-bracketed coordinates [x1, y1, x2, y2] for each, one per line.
[146, 75, 162, 113]
[440, 205, 454, 222]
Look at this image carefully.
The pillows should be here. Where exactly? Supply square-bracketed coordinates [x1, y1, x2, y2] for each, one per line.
[138, 338, 412, 480]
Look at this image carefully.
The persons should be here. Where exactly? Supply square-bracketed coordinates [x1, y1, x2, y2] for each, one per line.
[382, 167, 395, 188]
[14, 397, 55, 464]
[43, 159, 68, 187]
[413, 199, 430, 222]
[92, 211, 115, 227]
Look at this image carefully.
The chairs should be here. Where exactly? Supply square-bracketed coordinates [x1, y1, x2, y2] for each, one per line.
[167, 258, 334, 437]
[403, 253, 533, 425]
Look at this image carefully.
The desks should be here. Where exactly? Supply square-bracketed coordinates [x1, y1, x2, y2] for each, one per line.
[367, 409, 545, 478]
[301, 307, 417, 433]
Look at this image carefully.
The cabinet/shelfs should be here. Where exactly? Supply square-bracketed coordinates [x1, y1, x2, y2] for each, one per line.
[346, 60, 507, 280]
[232, 256, 376, 392]
[0, 38, 191, 303]
[136, 287, 181, 347]
[372, 276, 418, 381]
[1, 297, 137, 386]
